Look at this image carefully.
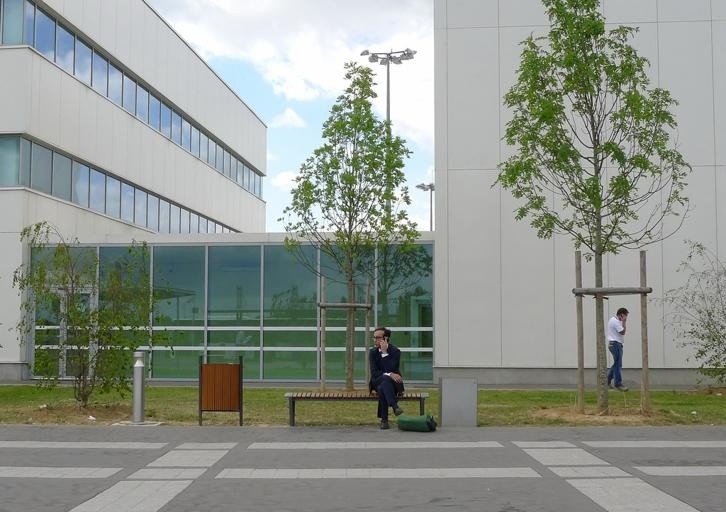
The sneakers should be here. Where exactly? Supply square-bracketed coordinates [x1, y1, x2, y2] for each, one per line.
[608, 384, 614, 388]
[615, 384, 629, 391]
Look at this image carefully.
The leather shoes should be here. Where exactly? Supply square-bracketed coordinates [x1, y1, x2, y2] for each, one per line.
[394, 408, 403, 416]
[381, 420, 388, 429]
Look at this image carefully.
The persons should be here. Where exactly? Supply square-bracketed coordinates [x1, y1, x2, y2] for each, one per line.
[366, 325, 406, 431]
[606, 307, 630, 393]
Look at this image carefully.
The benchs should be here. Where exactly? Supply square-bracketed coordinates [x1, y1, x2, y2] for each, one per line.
[284, 389, 429, 428]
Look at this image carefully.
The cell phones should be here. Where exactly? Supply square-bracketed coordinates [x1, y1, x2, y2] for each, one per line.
[621, 315, 623, 318]
[384, 337, 388, 343]
